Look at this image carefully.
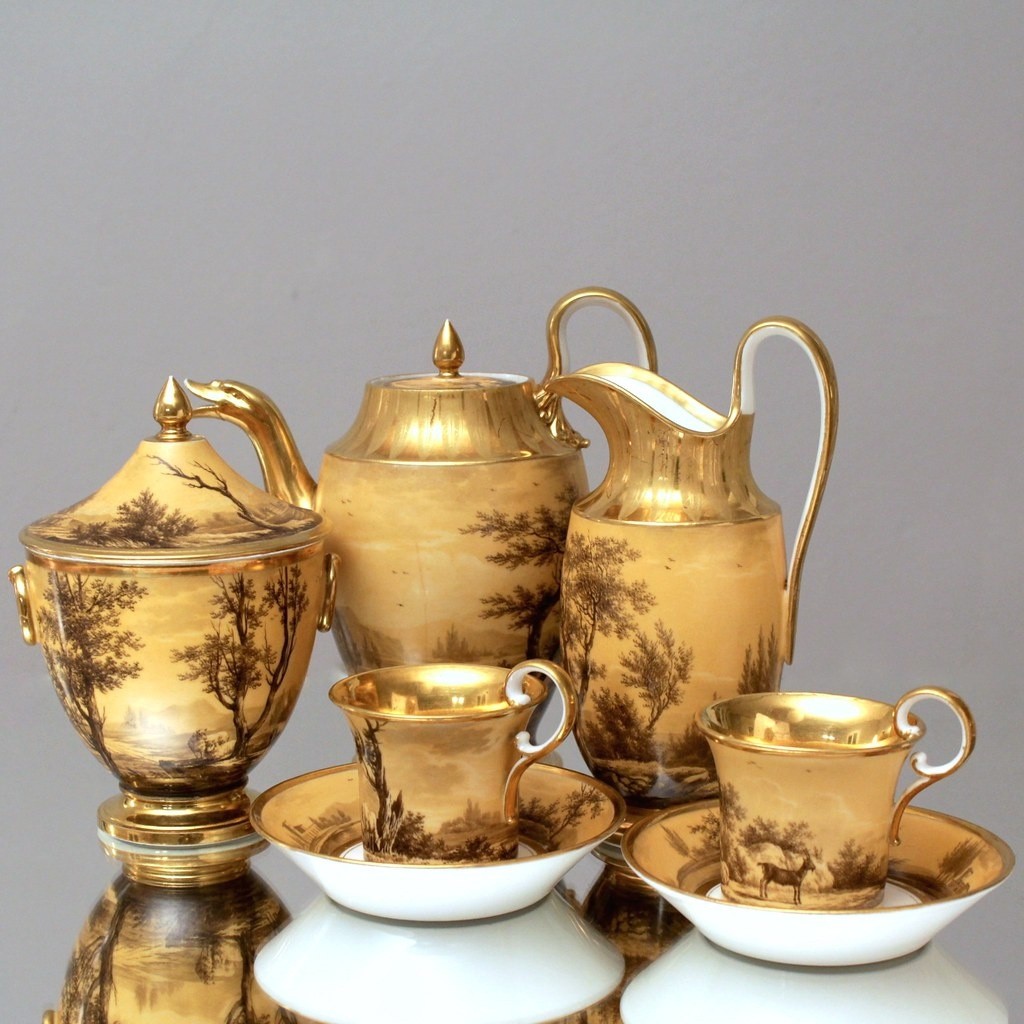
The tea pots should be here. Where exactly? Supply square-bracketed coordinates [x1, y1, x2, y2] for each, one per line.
[182, 289, 657, 729]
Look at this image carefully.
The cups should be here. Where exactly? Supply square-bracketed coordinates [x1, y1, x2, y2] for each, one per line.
[694, 690, 975, 903]
[331, 659, 574, 860]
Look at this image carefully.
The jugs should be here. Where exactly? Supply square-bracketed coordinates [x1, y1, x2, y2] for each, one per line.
[14, 382, 341, 851]
[42, 850, 293, 1024]
[546, 319, 838, 808]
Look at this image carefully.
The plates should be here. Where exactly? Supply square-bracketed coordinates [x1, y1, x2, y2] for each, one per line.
[620, 928, 1011, 1024]
[248, 761, 619, 923]
[621, 797, 1015, 969]
[254, 898, 621, 1024]
